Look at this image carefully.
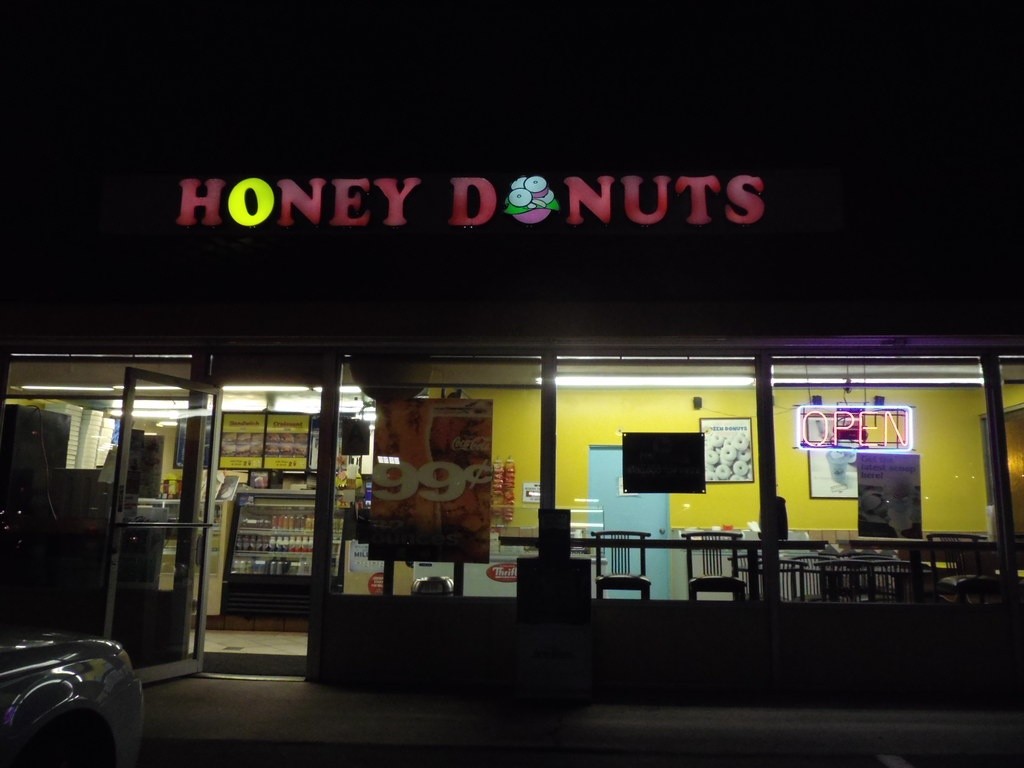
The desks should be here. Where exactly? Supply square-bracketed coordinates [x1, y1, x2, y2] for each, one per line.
[850, 539, 997, 604]
[499, 536, 829, 549]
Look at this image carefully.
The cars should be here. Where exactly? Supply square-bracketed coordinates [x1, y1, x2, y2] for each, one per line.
[0, 622, 145, 768]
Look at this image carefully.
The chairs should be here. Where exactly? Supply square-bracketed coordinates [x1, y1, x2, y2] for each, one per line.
[592, 530, 651, 600]
[681, 532, 747, 602]
[728, 533, 1002, 604]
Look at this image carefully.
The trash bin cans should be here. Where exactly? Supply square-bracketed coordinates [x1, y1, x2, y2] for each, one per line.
[516, 558, 594, 698]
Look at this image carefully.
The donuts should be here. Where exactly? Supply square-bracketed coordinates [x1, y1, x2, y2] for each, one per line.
[705, 432, 752, 481]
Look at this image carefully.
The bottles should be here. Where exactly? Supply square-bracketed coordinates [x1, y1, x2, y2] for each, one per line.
[155, 493, 177, 499]
[364, 477, 372, 510]
[271, 514, 344, 534]
[232, 556, 337, 576]
[235, 535, 342, 552]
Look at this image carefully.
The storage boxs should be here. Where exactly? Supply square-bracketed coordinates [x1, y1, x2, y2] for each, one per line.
[44, 402, 115, 470]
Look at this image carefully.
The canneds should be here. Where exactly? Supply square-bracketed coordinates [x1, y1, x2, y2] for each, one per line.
[239, 560, 284, 575]
[235, 535, 270, 551]
